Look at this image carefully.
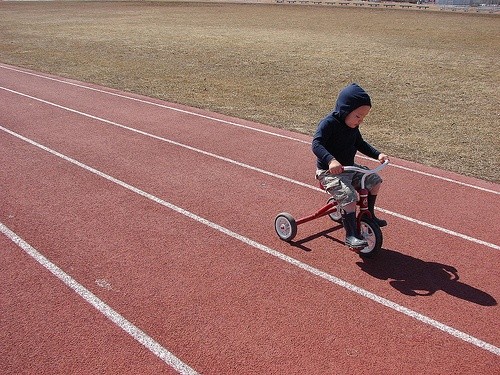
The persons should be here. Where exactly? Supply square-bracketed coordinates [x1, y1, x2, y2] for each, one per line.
[311, 81, 391, 247]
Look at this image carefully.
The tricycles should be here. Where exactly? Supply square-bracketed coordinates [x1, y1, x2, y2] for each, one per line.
[274, 158, 390, 259]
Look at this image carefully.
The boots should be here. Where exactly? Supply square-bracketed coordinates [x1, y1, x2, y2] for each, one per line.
[361, 195, 387, 227]
[341, 211, 369, 248]
